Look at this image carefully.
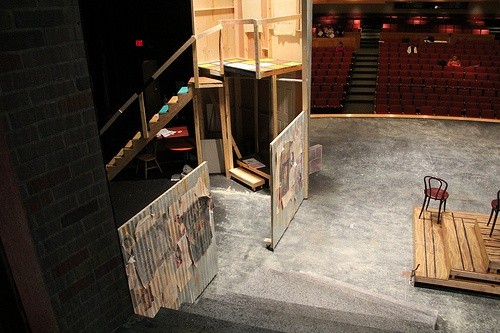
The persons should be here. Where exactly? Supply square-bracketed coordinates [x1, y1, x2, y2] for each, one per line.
[316, 23, 334, 38]
[448, 56, 461, 66]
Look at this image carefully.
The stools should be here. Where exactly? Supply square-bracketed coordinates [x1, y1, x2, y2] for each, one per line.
[135, 153, 163, 182]
[166, 142, 193, 174]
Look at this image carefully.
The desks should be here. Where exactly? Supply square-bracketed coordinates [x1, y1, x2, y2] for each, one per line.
[150, 126, 189, 166]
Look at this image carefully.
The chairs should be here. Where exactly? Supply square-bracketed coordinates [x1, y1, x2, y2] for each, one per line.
[418, 176, 449, 224]
[374, 16, 500, 120]
[311, 15, 364, 114]
[487, 190, 500, 236]
